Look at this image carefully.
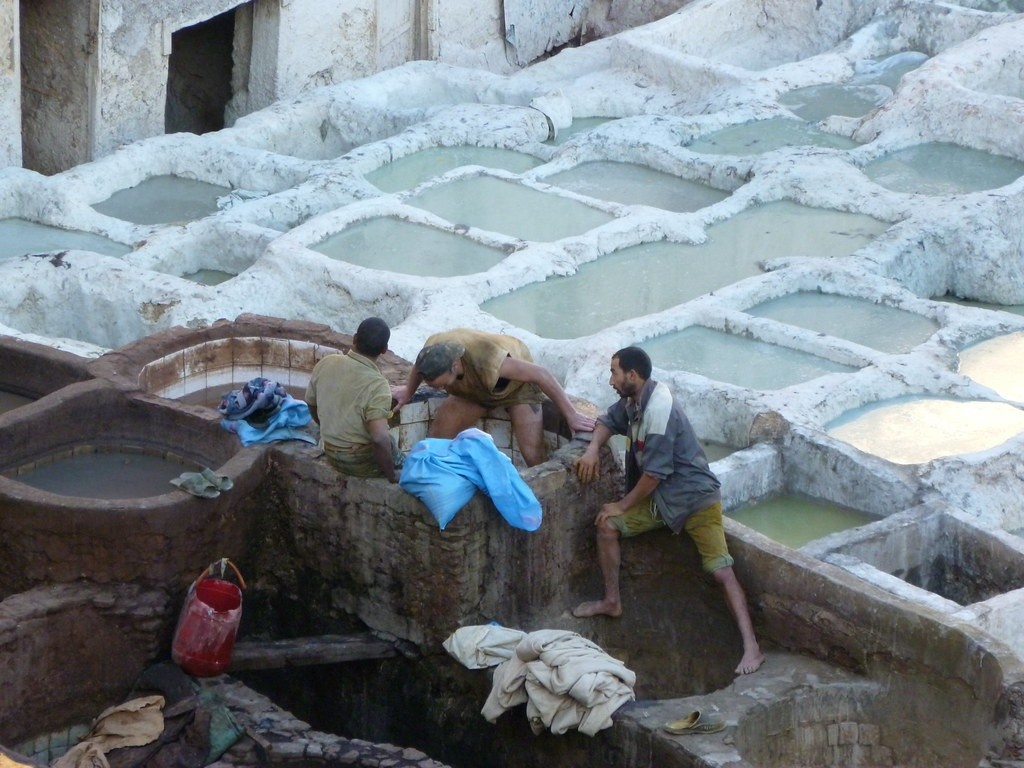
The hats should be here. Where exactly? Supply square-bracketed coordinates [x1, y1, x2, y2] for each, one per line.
[416, 339, 465, 379]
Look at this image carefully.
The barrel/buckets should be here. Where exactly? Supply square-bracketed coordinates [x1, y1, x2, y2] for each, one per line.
[171, 559, 249, 677]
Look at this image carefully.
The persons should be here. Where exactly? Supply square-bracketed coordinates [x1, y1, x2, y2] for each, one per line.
[304, 318, 408, 483]
[391, 328, 597, 467]
[572, 347, 766, 673]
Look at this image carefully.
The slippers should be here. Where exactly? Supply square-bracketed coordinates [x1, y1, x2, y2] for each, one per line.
[247, 411, 268, 428]
[665, 710, 728, 734]
[266, 404, 280, 416]
[169, 475, 219, 498]
[180, 472, 233, 491]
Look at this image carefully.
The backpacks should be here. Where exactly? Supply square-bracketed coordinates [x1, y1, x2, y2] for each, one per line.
[218, 376, 286, 420]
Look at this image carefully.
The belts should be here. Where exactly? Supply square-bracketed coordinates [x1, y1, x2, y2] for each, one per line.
[324, 444, 350, 452]
